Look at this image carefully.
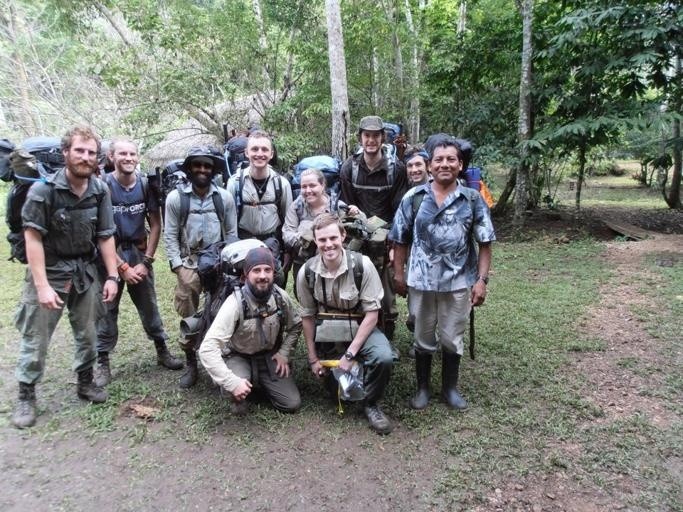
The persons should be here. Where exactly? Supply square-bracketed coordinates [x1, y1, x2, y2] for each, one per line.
[13, 128, 121, 428]
[387, 133, 495, 414]
[340, 116, 410, 362]
[92, 135, 183, 387]
[164, 145, 238, 388]
[402, 145, 430, 186]
[198, 248, 304, 412]
[455, 139, 494, 209]
[296, 214, 395, 436]
[227, 131, 295, 288]
[281, 168, 366, 302]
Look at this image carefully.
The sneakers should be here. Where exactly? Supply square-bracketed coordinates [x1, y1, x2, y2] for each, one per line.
[366, 404, 390, 431]
[231, 398, 248, 414]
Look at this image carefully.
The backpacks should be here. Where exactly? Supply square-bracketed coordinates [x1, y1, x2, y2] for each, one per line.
[223, 130, 285, 238]
[99, 139, 149, 250]
[299, 217, 386, 344]
[193, 237, 285, 362]
[342, 122, 407, 211]
[410, 165, 494, 245]
[6, 135, 106, 265]
[162, 161, 230, 253]
[286, 154, 341, 226]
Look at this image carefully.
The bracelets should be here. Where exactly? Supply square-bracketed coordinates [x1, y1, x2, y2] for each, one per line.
[117, 262, 129, 273]
[306, 357, 319, 364]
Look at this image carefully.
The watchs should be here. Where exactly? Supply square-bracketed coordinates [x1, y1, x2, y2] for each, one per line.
[343, 351, 356, 361]
[476, 276, 489, 284]
[105, 275, 121, 284]
[141, 255, 155, 266]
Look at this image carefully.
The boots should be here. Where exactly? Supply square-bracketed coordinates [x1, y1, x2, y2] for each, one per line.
[77, 368, 106, 403]
[441, 353, 468, 411]
[180, 349, 198, 386]
[155, 340, 184, 370]
[96, 351, 112, 389]
[414, 350, 434, 409]
[14, 381, 36, 428]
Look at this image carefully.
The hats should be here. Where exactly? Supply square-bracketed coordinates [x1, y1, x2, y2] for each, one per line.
[176, 145, 226, 174]
[358, 116, 384, 136]
[243, 248, 276, 276]
